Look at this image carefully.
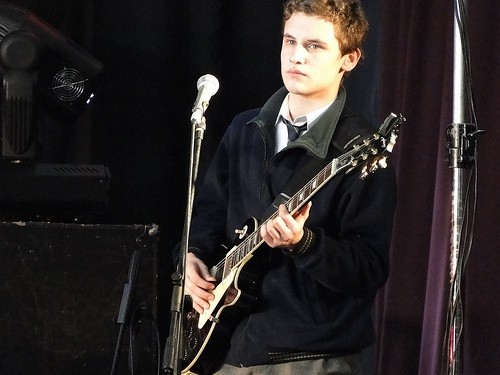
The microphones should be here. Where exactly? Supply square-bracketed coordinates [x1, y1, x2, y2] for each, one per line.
[190, 73, 219, 124]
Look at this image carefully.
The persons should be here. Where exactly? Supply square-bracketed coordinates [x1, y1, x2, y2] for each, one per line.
[172, 0, 396, 375]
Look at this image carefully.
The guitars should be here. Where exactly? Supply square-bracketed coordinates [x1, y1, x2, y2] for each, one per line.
[178, 112, 406, 375]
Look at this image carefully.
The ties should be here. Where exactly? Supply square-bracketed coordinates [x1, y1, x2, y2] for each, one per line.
[282, 116, 307, 147]
